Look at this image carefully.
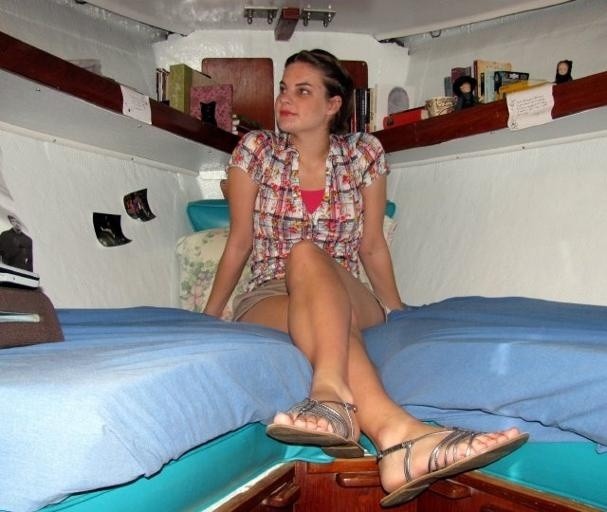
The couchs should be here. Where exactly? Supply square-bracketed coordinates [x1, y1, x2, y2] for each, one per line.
[0, 292, 607, 511]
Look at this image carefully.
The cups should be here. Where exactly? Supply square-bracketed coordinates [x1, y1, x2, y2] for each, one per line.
[425, 97, 456, 118]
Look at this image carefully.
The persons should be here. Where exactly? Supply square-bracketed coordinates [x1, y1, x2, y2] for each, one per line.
[202, 48, 529, 507]
[553, 60, 572, 84]
[0, 215, 32, 272]
[135, 200, 150, 220]
[101, 214, 120, 246]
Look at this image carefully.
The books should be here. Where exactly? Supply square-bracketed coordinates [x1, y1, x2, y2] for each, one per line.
[155, 68, 167, 103]
[443, 59, 546, 109]
[347, 85, 376, 133]
[190, 84, 233, 132]
[382, 106, 429, 129]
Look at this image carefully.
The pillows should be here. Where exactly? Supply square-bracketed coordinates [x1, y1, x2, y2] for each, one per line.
[177, 199, 397, 323]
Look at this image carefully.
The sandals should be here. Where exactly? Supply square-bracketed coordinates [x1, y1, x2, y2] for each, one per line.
[264, 397, 365, 459]
[376, 426, 531, 509]
